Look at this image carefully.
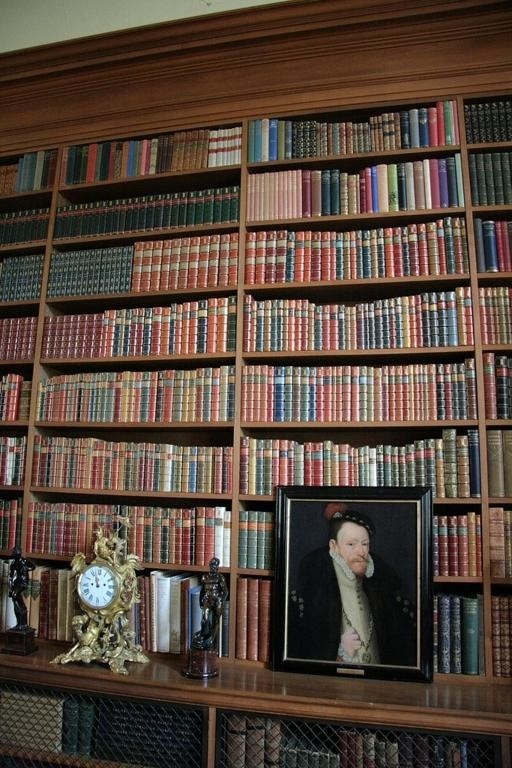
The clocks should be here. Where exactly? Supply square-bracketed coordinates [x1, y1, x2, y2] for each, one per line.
[48, 514, 150, 675]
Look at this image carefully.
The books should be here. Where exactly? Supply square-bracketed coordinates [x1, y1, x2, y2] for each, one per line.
[244, 101, 512, 221]
[242, 216, 512, 352]
[3, 364, 235, 493]
[3, 233, 240, 361]
[3, 691, 469, 766]
[2, 125, 242, 246]
[427, 508, 512, 678]
[1, 500, 232, 657]
[234, 510, 275, 662]
[240, 353, 512, 499]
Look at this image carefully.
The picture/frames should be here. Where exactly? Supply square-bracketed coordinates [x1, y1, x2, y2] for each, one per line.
[268, 486, 435, 683]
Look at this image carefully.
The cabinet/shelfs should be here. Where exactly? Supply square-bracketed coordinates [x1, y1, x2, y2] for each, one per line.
[1, 638, 511, 767]
[0, 147, 61, 556]
[456, 96, 512, 677]
[21, 124, 246, 661]
[233, 97, 490, 677]
